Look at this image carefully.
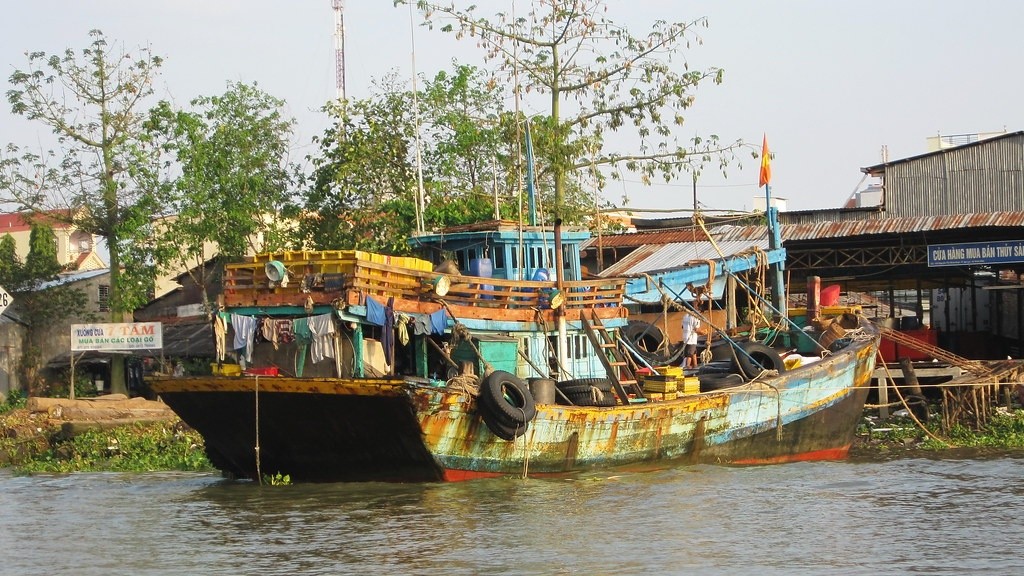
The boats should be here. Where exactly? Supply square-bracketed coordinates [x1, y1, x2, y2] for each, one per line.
[136, 0, 888, 486]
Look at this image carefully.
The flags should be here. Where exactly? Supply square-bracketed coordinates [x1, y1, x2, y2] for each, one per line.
[759, 133, 771, 187]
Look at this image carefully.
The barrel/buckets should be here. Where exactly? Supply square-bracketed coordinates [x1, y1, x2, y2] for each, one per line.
[527, 376, 556, 405]
[433, 256, 553, 310]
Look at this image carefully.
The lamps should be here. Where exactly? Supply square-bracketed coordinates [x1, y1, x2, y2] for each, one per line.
[420, 274, 452, 297]
[548, 289, 566, 309]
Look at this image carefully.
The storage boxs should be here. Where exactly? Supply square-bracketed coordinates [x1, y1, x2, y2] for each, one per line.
[815, 322, 848, 359]
[247, 366, 279, 378]
[253, 248, 433, 299]
[797, 331, 818, 355]
[210, 363, 242, 377]
[635, 365, 700, 402]
[792, 315, 808, 331]
[774, 334, 790, 348]
[811, 314, 836, 331]
[897, 329, 938, 360]
[877, 332, 896, 363]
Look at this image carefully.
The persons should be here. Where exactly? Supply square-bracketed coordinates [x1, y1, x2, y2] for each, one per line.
[681, 305, 705, 370]
